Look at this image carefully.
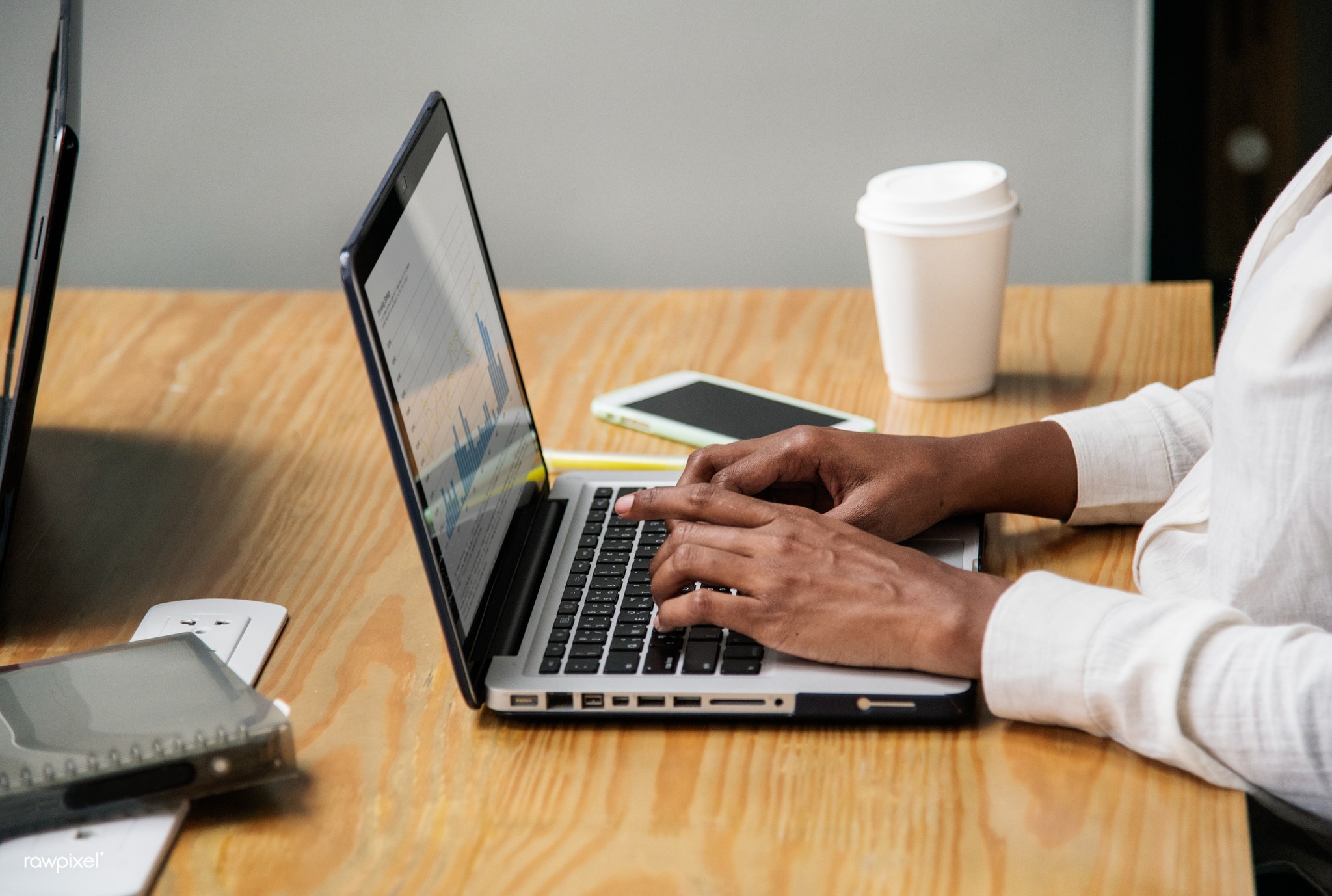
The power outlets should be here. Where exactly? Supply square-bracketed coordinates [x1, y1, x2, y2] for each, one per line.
[0, 595, 291, 896]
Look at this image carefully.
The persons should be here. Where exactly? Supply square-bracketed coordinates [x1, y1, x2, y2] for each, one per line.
[614, 137, 1332, 882]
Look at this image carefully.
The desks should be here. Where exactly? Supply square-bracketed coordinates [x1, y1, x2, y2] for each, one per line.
[0, 279, 1259, 896]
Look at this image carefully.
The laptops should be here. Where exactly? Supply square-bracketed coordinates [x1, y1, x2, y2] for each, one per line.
[339, 90, 987, 727]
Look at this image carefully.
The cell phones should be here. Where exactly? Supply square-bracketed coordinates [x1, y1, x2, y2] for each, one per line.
[589, 367, 876, 450]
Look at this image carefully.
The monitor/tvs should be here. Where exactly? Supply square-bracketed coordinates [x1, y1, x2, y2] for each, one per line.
[0, 0, 84, 498]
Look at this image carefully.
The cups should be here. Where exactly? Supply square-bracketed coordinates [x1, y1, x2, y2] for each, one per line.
[855, 160, 1021, 401]
